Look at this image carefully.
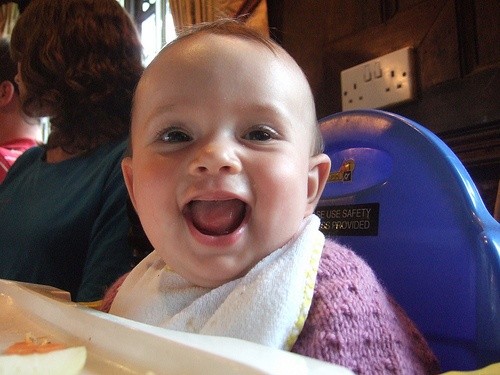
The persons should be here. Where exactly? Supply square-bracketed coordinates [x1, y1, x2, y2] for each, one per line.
[0, 35, 43, 184]
[0, 0, 154, 308]
[98, 17, 442, 374]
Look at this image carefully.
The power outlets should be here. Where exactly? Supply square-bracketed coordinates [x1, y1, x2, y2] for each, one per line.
[341, 46, 417, 112]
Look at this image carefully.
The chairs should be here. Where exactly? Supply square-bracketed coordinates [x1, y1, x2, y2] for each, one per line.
[317, 108, 500, 373]
